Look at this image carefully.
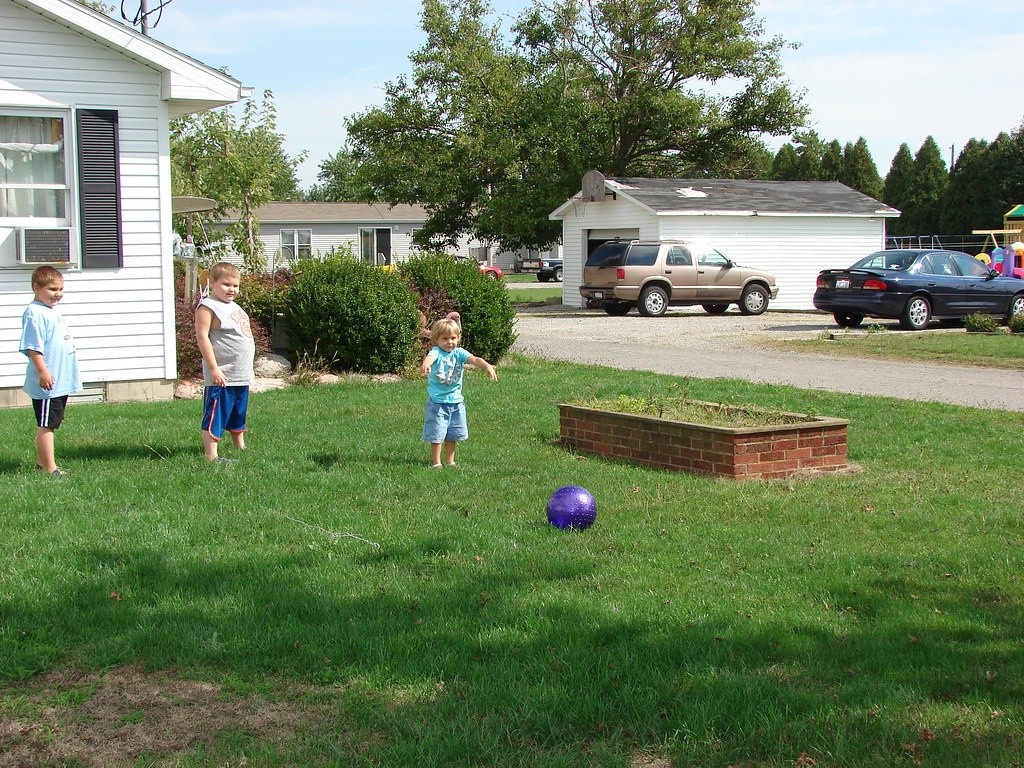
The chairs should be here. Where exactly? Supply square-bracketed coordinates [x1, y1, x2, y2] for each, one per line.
[674, 257, 688, 265]
[933, 263, 944, 274]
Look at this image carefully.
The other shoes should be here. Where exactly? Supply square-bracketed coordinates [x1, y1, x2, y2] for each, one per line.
[213, 457, 225, 463]
[51, 469, 65, 476]
[432, 464, 442, 467]
[35, 463, 43, 470]
[450, 462, 455, 466]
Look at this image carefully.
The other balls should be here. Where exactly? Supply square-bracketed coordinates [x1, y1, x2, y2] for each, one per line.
[546, 486, 597, 530]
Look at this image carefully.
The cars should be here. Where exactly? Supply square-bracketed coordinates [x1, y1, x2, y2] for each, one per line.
[812, 249, 1024, 331]
[454, 255, 502, 281]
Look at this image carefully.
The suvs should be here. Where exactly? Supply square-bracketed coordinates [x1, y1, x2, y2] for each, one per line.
[579, 238, 780, 318]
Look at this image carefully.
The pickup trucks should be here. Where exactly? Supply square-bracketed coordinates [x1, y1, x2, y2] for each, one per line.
[521, 257, 564, 282]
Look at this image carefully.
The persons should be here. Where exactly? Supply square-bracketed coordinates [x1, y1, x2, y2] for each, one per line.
[419, 319, 497, 468]
[172, 227, 196, 258]
[195, 262, 256, 462]
[18, 266, 83, 476]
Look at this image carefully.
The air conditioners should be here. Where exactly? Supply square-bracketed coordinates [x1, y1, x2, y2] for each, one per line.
[15, 226, 78, 265]
